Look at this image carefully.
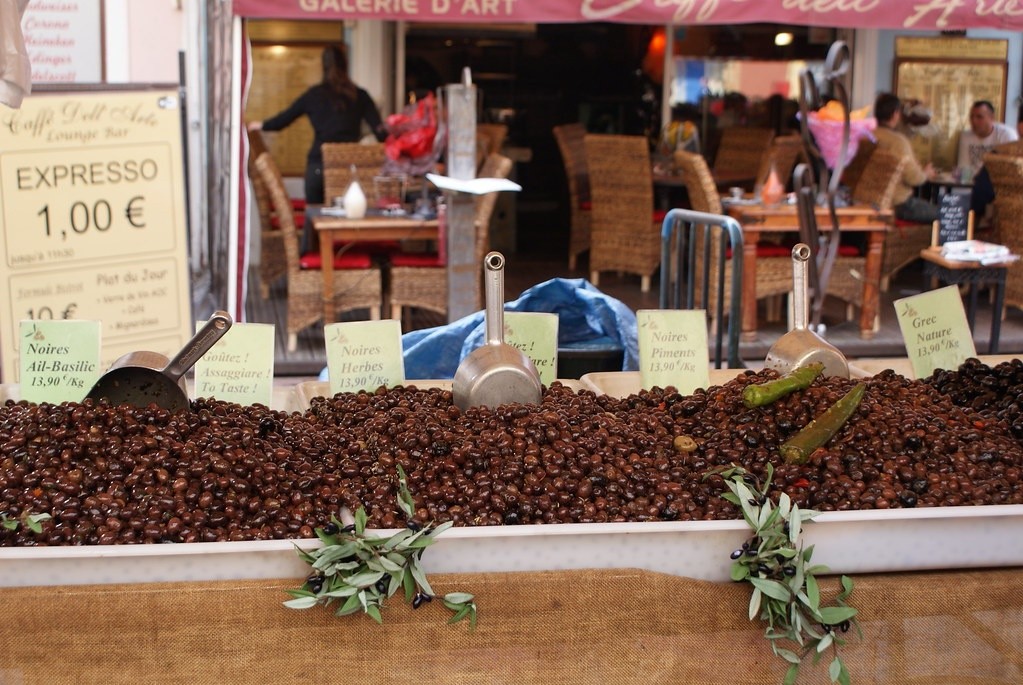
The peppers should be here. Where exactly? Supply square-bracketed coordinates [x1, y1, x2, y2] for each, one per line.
[741, 360, 865, 463]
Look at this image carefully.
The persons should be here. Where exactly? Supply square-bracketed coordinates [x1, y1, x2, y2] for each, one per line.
[958, 101, 1018, 171]
[247, 45, 389, 259]
[969, 104, 1023, 216]
[716, 91, 801, 132]
[869, 93, 938, 223]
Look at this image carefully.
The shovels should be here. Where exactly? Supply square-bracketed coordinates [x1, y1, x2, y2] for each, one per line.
[453, 250, 542, 409]
[763, 244, 850, 380]
[79, 310, 233, 414]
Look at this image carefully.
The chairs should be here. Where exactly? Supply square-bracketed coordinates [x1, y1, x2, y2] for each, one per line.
[243, 106, 1023, 367]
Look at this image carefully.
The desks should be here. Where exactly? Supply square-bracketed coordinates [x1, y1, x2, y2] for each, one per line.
[916, 178, 975, 210]
[718, 190, 896, 340]
[652, 163, 757, 211]
[304, 204, 446, 326]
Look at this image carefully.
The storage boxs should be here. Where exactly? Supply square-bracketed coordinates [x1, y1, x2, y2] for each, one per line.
[0, 491, 1022, 582]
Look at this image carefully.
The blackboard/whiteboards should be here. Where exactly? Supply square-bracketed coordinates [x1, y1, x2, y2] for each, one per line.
[931, 186, 974, 296]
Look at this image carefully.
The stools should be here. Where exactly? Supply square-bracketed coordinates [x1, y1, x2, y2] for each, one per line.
[922, 237, 1012, 356]
